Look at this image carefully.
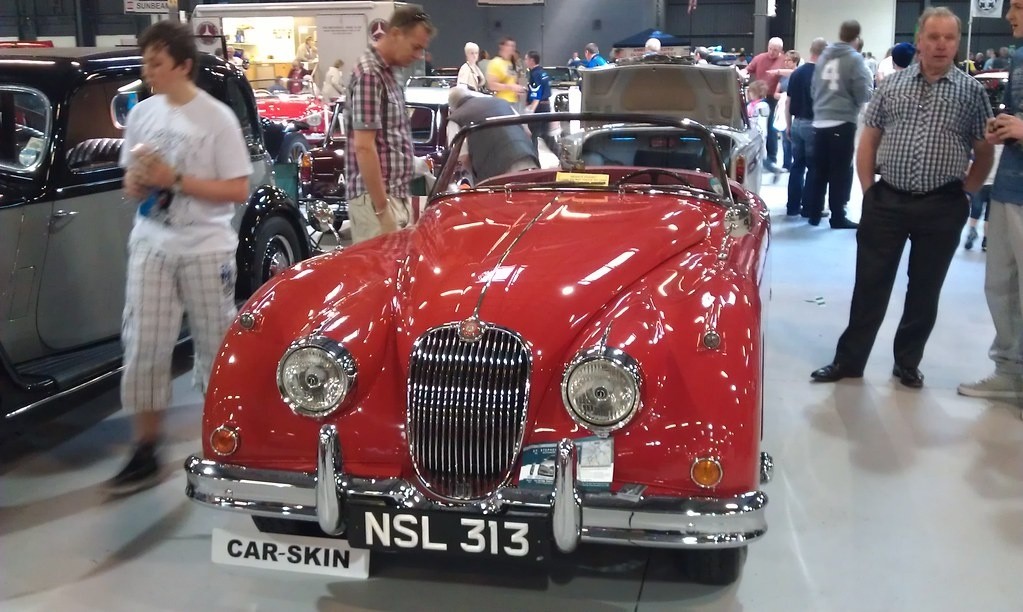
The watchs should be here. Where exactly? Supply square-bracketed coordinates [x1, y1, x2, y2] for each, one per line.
[169, 174, 183, 196]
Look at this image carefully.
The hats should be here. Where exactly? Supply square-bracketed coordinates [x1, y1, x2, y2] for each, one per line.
[893, 43, 915, 67]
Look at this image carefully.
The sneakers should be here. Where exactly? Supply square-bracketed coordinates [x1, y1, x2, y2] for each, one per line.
[958, 370, 1023, 398]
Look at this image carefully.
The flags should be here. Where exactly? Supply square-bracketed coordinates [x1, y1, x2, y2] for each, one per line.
[687, 0, 697, 16]
[970, 0, 1004, 19]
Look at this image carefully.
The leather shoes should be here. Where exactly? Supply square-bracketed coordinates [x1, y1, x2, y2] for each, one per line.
[893, 364, 924, 387]
[811, 363, 847, 381]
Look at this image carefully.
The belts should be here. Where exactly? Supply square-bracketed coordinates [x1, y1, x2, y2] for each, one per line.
[881, 178, 955, 198]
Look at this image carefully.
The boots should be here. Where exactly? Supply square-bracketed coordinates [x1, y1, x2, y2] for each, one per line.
[768, 162, 782, 183]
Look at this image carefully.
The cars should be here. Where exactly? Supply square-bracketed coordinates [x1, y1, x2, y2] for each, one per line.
[0, 38, 535, 425]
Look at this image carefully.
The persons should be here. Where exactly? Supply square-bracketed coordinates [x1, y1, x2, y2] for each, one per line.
[97, 20, 257, 497]
[810, 9, 994, 388]
[965, 103, 1007, 251]
[456, 34, 564, 160]
[734, 50, 753, 69]
[693, 45, 713, 66]
[214, 44, 245, 70]
[642, 37, 662, 58]
[567, 41, 608, 92]
[743, 36, 801, 185]
[962, 45, 1010, 99]
[292, 34, 318, 70]
[956, 0, 1023, 403]
[343, 5, 437, 249]
[785, 19, 874, 229]
[321, 58, 347, 102]
[861, 43, 916, 88]
[609, 48, 626, 64]
[425, 51, 439, 86]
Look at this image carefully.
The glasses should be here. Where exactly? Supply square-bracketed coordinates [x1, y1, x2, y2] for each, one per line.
[398, 13, 428, 28]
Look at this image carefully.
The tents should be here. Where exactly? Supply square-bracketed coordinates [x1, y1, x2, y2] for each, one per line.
[611, 26, 691, 56]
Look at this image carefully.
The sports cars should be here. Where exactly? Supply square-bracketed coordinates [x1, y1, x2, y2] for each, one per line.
[560, 60, 765, 196]
[182, 108, 775, 586]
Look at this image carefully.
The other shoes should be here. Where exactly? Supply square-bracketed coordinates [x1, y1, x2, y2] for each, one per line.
[965, 230, 977, 248]
[830, 219, 859, 228]
[982, 239, 986, 251]
[103, 446, 163, 493]
[821, 211, 829, 217]
[808, 214, 821, 226]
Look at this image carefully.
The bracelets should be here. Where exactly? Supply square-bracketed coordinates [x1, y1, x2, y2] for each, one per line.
[778, 68, 780, 77]
[966, 190, 973, 201]
[374, 199, 391, 216]
